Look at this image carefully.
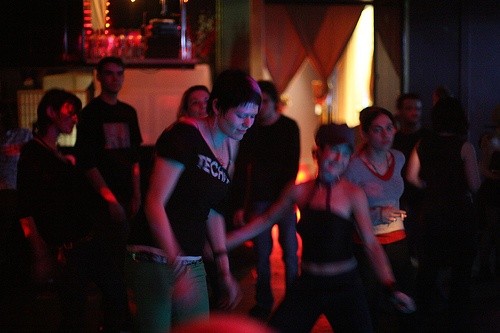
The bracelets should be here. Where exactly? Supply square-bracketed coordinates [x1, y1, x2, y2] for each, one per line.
[212, 247, 228, 258]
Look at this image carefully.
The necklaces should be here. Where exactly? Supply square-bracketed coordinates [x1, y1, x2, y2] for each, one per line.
[208, 127, 225, 166]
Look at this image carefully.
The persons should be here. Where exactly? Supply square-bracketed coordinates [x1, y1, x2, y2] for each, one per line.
[389, 94, 430, 247]
[202, 124, 416, 333]
[472, 103, 500, 287]
[120, 69, 262, 333]
[0, 87, 90, 333]
[177, 85, 211, 118]
[67, 56, 160, 333]
[347, 108, 425, 333]
[405, 96, 494, 284]
[231, 80, 298, 320]
